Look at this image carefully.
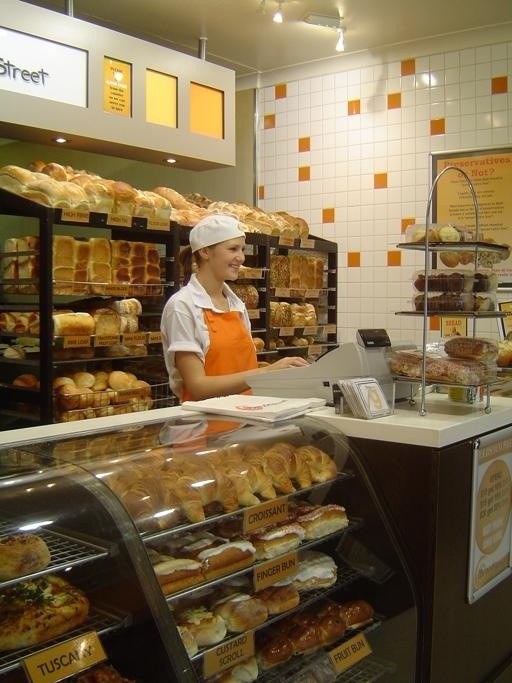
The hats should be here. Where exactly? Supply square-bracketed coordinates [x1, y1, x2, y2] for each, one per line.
[189, 215, 246, 253]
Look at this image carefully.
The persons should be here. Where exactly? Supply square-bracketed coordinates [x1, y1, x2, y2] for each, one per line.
[160, 214, 309, 403]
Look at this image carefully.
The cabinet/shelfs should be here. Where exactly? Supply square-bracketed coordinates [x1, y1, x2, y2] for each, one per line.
[0, 398, 421, 683]
[0, 158, 341, 422]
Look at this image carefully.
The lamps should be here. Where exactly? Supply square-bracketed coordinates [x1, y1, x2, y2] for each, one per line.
[336, 33, 343, 53]
[271, 5, 285, 23]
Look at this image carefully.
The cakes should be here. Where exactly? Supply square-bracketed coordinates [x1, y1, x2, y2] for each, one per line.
[0, 533, 51, 578]
[68, 665, 135, 683]
[1, 575, 89, 652]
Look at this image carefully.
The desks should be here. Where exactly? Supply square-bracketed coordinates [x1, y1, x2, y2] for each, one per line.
[248, 384, 512, 681]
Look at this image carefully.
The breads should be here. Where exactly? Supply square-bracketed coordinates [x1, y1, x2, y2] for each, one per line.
[201, 201, 267, 219]
[108, 371, 129, 390]
[274, 442, 295, 448]
[252, 338, 265, 352]
[303, 303, 317, 326]
[301, 255, 315, 296]
[215, 594, 267, 632]
[28, 161, 46, 172]
[29, 313, 40, 335]
[341, 601, 375, 630]
[278, 550, 337, 590]
[102, 182, 136, 214]
[173, 211, 203, 227]
[91, 391, 110, 407]
[121, 427, 161, 455]
[51, 439, 90, 467]
[111, 240, 131, 295]
[160, 463, 203, 505]
[132, 187, 155, 218]
[196, 541, 256, 580]
[84, 298, 141, 316]
[81, 407, 96, 419]
[270, 255, 290, 288]
[290, 255, 300, 296]
[181, 450, 228, 474]
[53, 235, 75, 295]
[164, 531, 213, 554]
[177, 625, 197, 659]
[143, 396, 154, 408]
[154, 558, 205, 594]
[263, 448, 312, 493]
[70, 176, 96, 190]
[231, 284, 259, 309]
[175, 466, 238, 523]
[75, 307, 121, 333]
[291, 607, 318, 625]
[53, 377, 76, 396]
[141, 191, 171, 219]
[136, 360, 170, 380]
[223, 443, 254, 463]
[13, 374, 38, 388]
[131, 381, 151, 396]
[289, 626, 322, 657]
[147, 244, 161, 294]
[78, 388, 94, 409]
[34, 172, 57, 184]
[249, 579, 299, 615]
[392, 350, 448, 378]
[56, 181, 89, 210]
[223, 462, 276, 506]
[105, 387, 128, 404]
[121, 474, 182, 532]
[151, 555, 173, 567]
[120, 315, 139, 334]
[291, 304, 304, 326]
[270, 302, 280, 327]
[126, 374, 136, 384]
[89, 370, 108, 381]
[55, 345, 148, 360]
[65, 164, 87, 178]
[154, 186, 187, 210]
[278, 210, 309, 240]
[297, 505, 349, 540]
[2, 238, 18, 294]
[280, 303, 291, 327]
[477, 233, 510, 268]
[297, 447, 338, 483]
[130, 243, 147, 294]
[496, 339, 512, 368]
[88, 238, 111, 295]
[165, 453, 185, 466]
[6, 345, 25, 360]
[318, 614, 346, 646]
[95, 406, 114, 416]
[441, 364, 478, 384]
[90, 173, 108, 181]
[270, 614, 311, 634]
[211, 657, 258, 683]
[252, 525, 306, 561]
[1, 165, 39, 195]
[176, 536, 226, 557]
[240, 216, 279, 234]
[216, 519, 246, 536]
[121, 456, 161, 470]
[146, 546, 156, 558]
[412, 227, 477, 244]
[279, 498, 319, 525]
[270, 212, 297, 238]
[52, 312, 95, 335]
[94, 462, 120, 482]
[444, 337, 499, 361]
[23, 180, 73, 209]
[270, 336, 314, 348]
[243, 443, 273, 462]
[56, 384, 79, 411]
[220, 576, 249, 594]
[111, 469, 144, 494]
[260, 636, 294, 668]
[72, 241, 89, 295]
[113, 404, 131, 415]
[416, 272, 499, 294]
[312, 599, 338, 622]
[18, 237, 40, 295]
[0, 312, 21, 333]
[73, 372, 95, 390]
[174, 588, 221, 605]
[254, 629, 277, 654]
[41, 163, 66, 182]
[14, 313, 32, 335]
[315, 258, 324, 289]
[187, 202, 200, 210]
[60, 412, 85, 422]
[90, 434, 120, 462]
[91, 381, 107, 391]
[83, 181, 114, 213]
[466, 361, 491, 383]
[415, 295, 495, 311]
[10, 446, 45, 467]
[441, 252, 475, 268]
[128, 397, 146, 412]
[173, 605, 227, 647]
